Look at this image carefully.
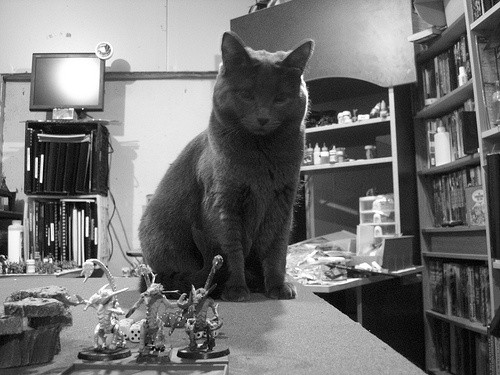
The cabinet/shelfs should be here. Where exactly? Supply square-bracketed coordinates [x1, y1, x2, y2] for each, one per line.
[407, 0, 500, 375]
[230, 0, 426, 369]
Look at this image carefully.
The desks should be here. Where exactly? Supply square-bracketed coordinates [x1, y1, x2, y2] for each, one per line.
[0, 269, 427, 375]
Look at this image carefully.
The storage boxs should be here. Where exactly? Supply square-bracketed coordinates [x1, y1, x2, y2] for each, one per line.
[18, 195, 108, 271]
[24, 119, 108, 195]
[357, 196, 396, 248]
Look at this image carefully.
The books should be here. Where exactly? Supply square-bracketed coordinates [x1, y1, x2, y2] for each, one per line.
[421, 0, 500, 375]
[24, 128, 98, 267]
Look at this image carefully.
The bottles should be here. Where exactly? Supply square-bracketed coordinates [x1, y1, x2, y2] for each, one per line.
[304, 142, 314, 166]
[379, 100, 388, 117]
[491, 80, 500, 125]
[314, 142, 320, 165]
[26, 259, 36, 274]
[458, 66, 468, 87]
[8, 220, 24, 265]
[433, 126, 452, 166]
[364, 145, 373, 159]
[370, 102, 381, 118]
[336, 150, 344, 163]
[321, 143, 330, 164]
[372, 146, 376, 159]
[328, 145, 337, 163]
[337, 110, 351, 124]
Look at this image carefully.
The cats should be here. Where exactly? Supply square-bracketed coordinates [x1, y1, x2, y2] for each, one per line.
[137, 30, 315, 301]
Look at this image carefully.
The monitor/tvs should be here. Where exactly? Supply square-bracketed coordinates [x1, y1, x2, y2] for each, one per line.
[29, 53, 103, 120]
